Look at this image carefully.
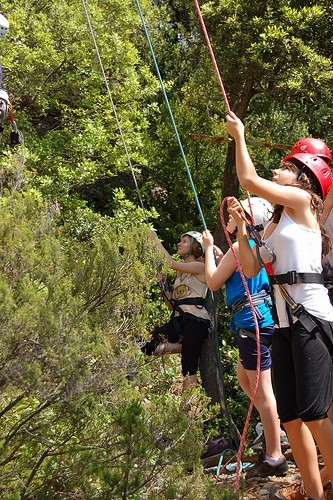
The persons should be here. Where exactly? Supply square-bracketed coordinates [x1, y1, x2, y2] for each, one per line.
[224, 112, 333, 500]
[141, 231, 209, 412]
[202, 197, 288, 479]
[275, 465, 331, 500]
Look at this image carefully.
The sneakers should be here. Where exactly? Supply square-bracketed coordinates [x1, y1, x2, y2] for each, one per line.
[269, 479, 314, 500]
[323, 481, 333, 500]
[245, 451, 290, 484]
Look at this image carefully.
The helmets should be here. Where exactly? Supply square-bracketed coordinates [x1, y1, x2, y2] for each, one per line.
[240, 197, 275, 228]
[282, 153, 333, 201]
[181, 230, 205, 256]
[292, 138, 332, 166]
[0, 14, 10, 38]
